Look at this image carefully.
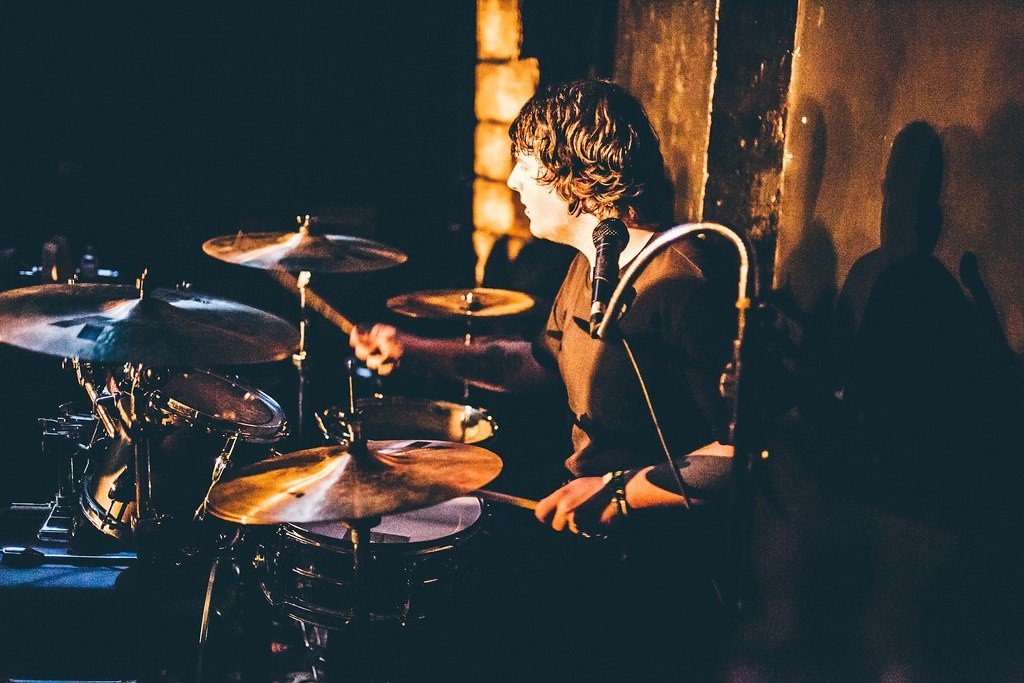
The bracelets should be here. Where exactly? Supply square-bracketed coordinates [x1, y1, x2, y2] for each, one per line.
[614, 468, 630, 519]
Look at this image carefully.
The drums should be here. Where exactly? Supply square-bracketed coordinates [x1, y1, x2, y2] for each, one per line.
[78, 367, 290, 546]
[254, 492, 492, 633]
[315, 397, 499, 456]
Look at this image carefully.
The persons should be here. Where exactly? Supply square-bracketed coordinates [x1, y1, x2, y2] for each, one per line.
[347, 75, 757, 683]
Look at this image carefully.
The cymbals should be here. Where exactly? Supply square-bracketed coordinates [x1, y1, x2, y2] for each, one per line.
[202, 224, 408, 274]
[0, 279, 302, 367]
[383, 287, 537, 319]
[205, 439, 504, 527]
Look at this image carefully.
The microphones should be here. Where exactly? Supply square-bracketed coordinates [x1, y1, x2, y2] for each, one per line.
[589, 218, 629, 339]
